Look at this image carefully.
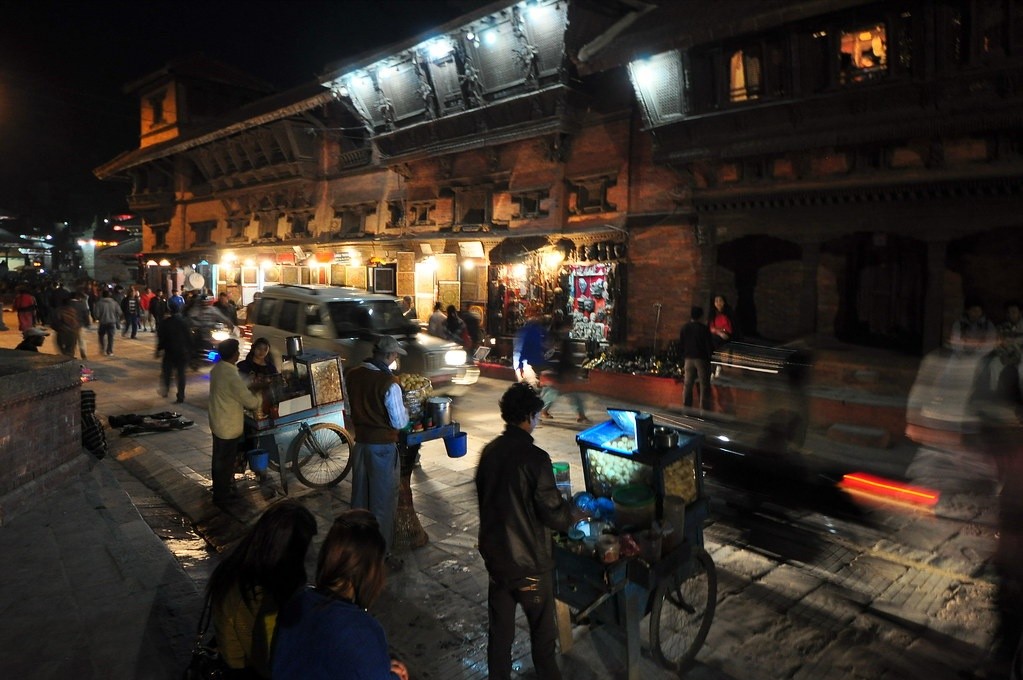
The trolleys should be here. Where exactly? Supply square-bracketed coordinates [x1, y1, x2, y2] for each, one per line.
[550, 407, 718, 670]
[240, 348, 353, 488]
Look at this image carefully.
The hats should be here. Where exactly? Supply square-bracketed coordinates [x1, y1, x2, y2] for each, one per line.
[374, 336, 407, 356]
[26, 328, 50, 336]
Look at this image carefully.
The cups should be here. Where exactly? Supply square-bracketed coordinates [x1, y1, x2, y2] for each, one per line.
[635, 413, 656, 453]
[597, 534, 620, 563]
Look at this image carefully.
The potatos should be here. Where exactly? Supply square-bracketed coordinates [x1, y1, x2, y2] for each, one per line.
[397, 373, 433, 417]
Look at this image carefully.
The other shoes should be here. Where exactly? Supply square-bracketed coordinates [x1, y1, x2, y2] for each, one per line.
[107, 353, 114, 356]
[384, 557, 402, 570]
[540, 411, 553, 418]
[157, 390, 167, 397]
[577, 417, 592, 424]
[131, 336, 137, 339]
[176, 393, 183, 403]
[212, 493, 244, 504]
[121, 333, 125, 337]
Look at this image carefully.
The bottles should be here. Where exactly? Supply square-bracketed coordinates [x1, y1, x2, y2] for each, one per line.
[611, 485, 655, 527]
[551, 462, 571, 504]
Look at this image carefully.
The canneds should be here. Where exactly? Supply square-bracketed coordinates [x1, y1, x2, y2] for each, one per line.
[582, 533, 619, 565]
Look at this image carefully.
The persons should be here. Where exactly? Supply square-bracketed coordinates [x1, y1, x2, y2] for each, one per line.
[272, 509, 408, 680]
[681, 296, 737, 413]
[201, 502, 317, 680]
[475, 383, 585, 680]
[0, 262, 276, 503]
[345, 334, 414, 568]
[512, 306, 594, 427]
[400, 295, 485, 366]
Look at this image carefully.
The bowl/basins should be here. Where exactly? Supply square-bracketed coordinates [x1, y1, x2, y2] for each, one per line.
[653, 427, 678, 449]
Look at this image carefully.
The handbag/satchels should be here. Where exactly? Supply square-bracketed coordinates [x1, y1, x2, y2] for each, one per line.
[191, 646, 226, 680]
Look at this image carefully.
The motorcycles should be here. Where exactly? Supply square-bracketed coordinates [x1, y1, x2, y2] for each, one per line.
[190, 316, 230, 371]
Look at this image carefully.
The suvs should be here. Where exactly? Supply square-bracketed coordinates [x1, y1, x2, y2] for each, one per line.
[244, 283, 480, 397]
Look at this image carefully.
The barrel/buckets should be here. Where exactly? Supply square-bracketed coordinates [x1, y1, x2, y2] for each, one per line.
[247, 438, 270, 472]
[444, 420, 467, 458]
[285, 336, 303, 357]
[427, 396, 452, 427]
